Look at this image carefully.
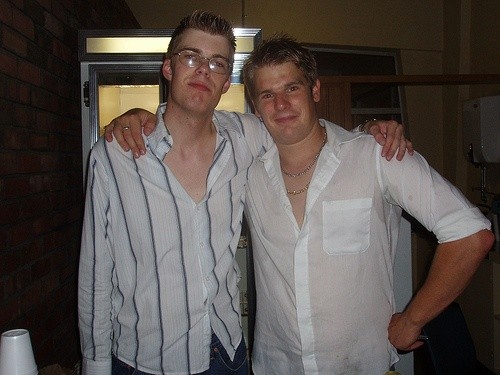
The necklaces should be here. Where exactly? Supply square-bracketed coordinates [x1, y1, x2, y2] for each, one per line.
[278, 129, 328, 177]
[285, 181, 310, 195]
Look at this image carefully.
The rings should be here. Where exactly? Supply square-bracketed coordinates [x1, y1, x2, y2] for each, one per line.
[122, 127, 131, 132]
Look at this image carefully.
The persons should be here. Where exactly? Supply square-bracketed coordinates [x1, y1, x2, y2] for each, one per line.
[104, 33, 497, 375]
[78, 9, 413, 374]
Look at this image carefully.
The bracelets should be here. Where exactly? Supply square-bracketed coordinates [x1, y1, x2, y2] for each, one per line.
[360, 117, 377, 134]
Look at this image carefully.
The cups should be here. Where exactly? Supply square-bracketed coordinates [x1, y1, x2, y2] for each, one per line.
[0, 329, 38, 375]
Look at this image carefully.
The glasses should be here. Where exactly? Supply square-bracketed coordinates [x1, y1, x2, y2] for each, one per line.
[170, 48, 233, 74]
